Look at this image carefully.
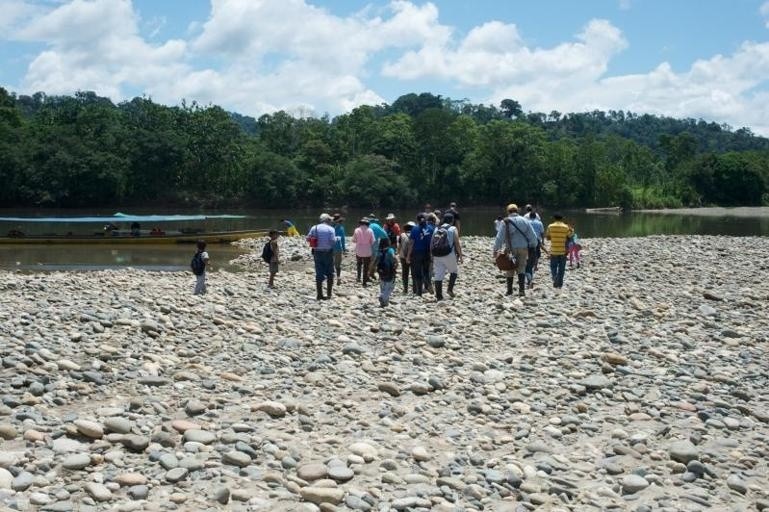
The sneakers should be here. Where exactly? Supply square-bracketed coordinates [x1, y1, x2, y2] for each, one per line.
[506, 291, 512, 296]
[447, 287, 454, 297]
[437, 292, 443, 300]
[427, 284, 434, 295]
[519, 288, 525, 296]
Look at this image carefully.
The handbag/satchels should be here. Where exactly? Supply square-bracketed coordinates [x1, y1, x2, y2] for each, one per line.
[496, 218, 519, 270]
[309, 225, 318, 247]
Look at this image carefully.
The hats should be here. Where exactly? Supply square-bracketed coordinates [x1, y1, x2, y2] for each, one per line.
[507, 203, 518, 211]
[368, 213, 379, 222]
[334, 213, 345, 222]
[526, 204, 532, 208]
[424, 204, 431, 208]
[386, 213, 395, 219]
[450, 202, 456, 207]
[319, 213, 334, 222]
[360, 216, 369, 223]
[404, 221, 416, 226]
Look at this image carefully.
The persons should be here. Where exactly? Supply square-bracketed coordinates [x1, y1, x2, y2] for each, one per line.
[444, 202, 460, 235]
[384, 213, 401, 249]
[427, 216, 439, 231]
[494, 216, 506, 232]
[351, 216, 377, 287]
[526, 212, 545, 271]
[430, 213, 463, 300]
[493, 204, 538, 296]
[366, 213, 390, 282]
[331, 213, 348, 285]
[546, 213, 575, 289]
[526, 236, 550, 286]
[306, 212, 338, 299]
[417, 203, 433, 218]
[568, 233, 582, 269]
[377, 237, 398, 307]
[426, 209, 442, 226]
[523, 203, 541, 220]
[191, 240, 211, 295]
[397, 221, 417, 295]
[262, 229, 281, 289]
[281, 219, 300, 237]
[406, 213, 434, 296]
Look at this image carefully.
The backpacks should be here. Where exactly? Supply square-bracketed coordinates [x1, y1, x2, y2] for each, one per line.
[191, 251, 205, 275]
[262, 240, 278, 264]
[430, 224, 455, 257]
